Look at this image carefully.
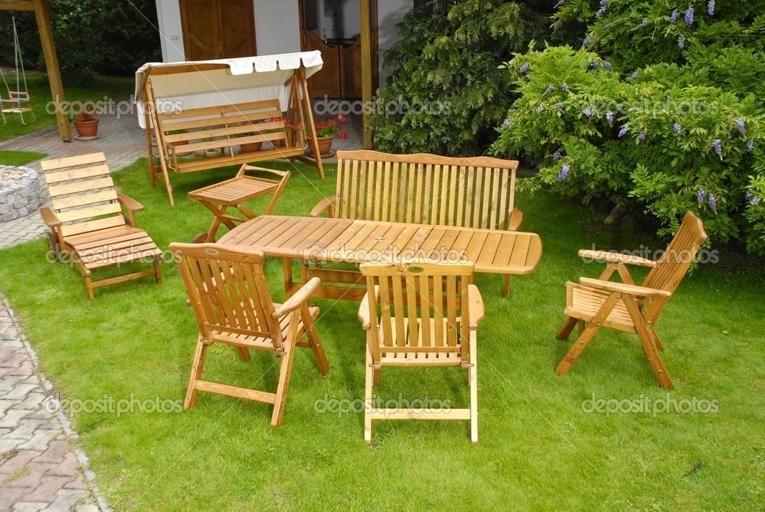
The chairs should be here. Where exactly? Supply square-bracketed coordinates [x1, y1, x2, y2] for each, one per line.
[168, 241, 329, 426]
[358, 260, 484, 445]
[40, 151, 163, 300]
[0, 91, 35, 125]
[554, 209, 707, 389]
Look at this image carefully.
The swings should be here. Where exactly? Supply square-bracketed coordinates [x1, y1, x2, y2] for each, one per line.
[0, 8, 36, 129]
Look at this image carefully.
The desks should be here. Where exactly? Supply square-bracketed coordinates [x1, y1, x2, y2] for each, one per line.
[215, 215, 543, 300]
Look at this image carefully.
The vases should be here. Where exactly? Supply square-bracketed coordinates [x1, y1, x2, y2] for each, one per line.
[240, 142, 262, 152]
[272, 139, 285, 148]
[308, 137, 335, 158]
[75, 120, 99, 140]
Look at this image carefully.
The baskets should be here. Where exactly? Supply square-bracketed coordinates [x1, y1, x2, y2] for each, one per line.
[307, 138, 332, 154]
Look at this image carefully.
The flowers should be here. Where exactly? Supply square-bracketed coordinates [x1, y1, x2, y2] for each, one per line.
[238, 121, 258, 136]
[74, 103, 95, 121]
[266, 118, 282, 131]
[305, 110, 348, 140]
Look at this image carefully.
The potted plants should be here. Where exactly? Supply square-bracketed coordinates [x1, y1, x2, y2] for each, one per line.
[202, 126, 224, 157]
[165, 129, 190, 156]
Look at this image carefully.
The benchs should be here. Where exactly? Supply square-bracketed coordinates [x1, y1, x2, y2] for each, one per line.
[134, 49, 325, 206]
[311, 150, 523, 298]
